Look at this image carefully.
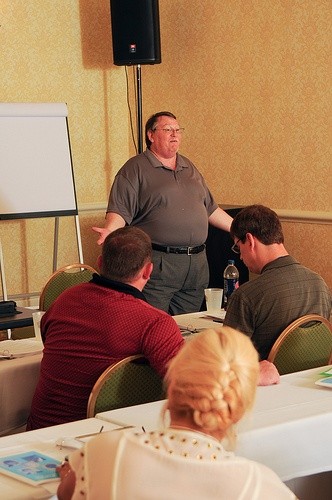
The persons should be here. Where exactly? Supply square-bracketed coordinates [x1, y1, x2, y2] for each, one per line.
[56, 327, 299, 500]
[223, 204, 330, 361]
[26, 226, 280, 431]
[92, 111, 234, 316]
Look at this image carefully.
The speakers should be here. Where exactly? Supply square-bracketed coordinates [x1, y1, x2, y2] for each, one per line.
[109, 0, 161, 66]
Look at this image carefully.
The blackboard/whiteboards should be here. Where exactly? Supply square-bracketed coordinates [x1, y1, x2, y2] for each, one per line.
[0, 101, 80, 221]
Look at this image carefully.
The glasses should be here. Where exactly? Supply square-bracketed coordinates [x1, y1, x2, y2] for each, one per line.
[231, 237, 243, 254]
[157, 128, 185, 132]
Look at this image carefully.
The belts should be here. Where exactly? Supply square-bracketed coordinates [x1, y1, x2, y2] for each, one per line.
[151, 243, 206, 255]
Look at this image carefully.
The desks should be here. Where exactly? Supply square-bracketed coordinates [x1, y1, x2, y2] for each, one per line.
[0, 364, 332, 500]
[0, 309, 227, 436]
[0, 305, 38, 341]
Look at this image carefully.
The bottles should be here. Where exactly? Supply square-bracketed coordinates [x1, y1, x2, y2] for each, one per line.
[223, 260, 239, 311]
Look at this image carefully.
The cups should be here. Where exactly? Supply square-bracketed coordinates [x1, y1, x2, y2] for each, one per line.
[32, 312, 46, 341]
[204, 288, 223, 315]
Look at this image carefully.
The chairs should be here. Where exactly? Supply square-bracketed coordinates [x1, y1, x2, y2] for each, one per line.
[268, 314, 332, 375]
[86, 354, 165, 418]
[39, 264, 100, 310]
